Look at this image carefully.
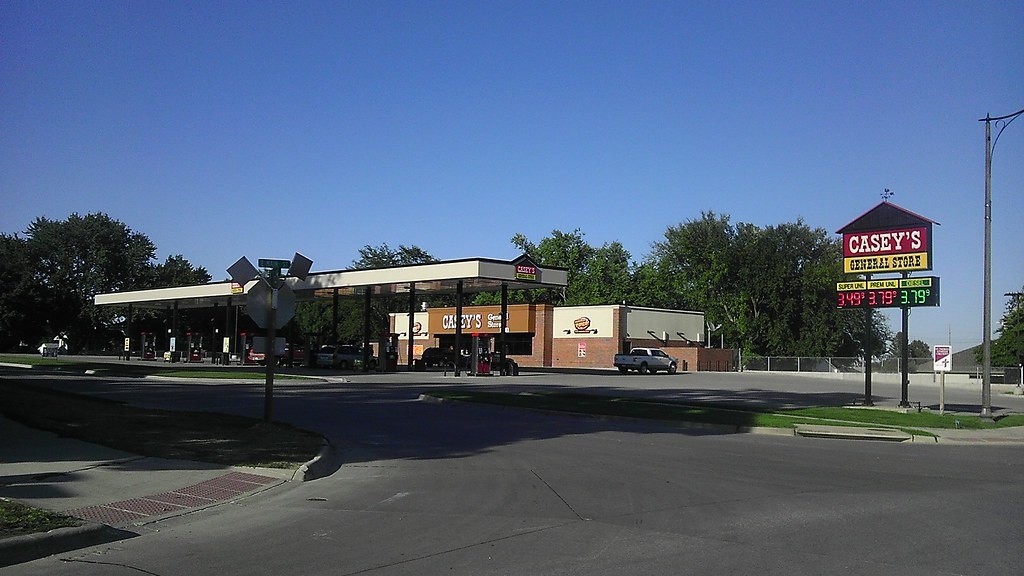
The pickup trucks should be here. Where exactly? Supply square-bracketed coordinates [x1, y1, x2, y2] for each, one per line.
[613, 348, 678, 375]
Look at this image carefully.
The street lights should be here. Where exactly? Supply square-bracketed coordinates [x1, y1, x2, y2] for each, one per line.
[1004, 292, 1024, 387]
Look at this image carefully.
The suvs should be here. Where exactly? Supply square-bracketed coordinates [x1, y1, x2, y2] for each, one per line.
[421, 347, 467, 368]
[317, 345, 379, 369]
[248, 343, 304, 366]
